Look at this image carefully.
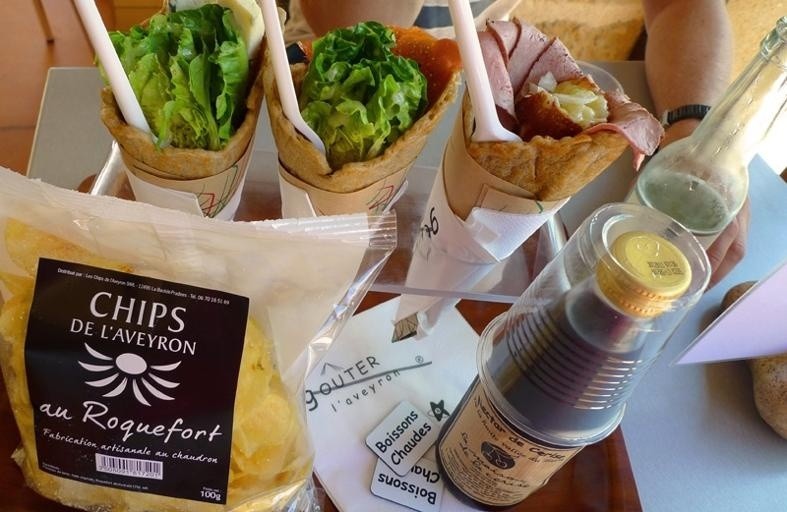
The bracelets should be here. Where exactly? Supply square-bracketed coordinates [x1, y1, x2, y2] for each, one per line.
[661, 102, 718, 132]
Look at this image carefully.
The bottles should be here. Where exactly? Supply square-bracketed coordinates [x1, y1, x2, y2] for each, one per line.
[624, 8, 787, 258]
[438, 226, 692, 511]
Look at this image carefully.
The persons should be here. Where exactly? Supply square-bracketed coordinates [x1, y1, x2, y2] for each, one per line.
[287, 0, 753, 302]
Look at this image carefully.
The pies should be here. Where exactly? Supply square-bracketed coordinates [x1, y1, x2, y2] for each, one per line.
[464, 79, 631, 201]
[265, 18, 457, 194]
[103, 33, 272, 179]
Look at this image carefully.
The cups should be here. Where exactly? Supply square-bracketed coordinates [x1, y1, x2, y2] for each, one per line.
[472, 196, 712, 450]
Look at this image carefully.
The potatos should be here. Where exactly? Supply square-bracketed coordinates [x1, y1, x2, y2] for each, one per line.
[720, 280, 787, 442]
